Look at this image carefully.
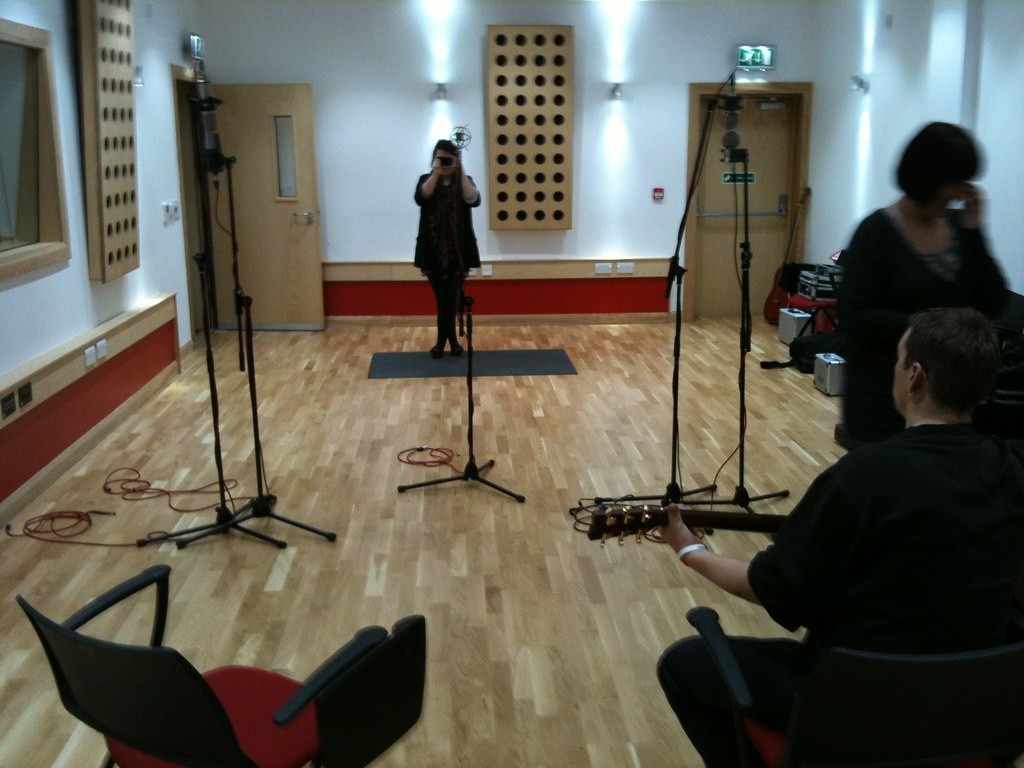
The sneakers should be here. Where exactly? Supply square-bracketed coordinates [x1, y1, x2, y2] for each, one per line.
[451, 342, 465, 355]
[430, 342, 444, 358]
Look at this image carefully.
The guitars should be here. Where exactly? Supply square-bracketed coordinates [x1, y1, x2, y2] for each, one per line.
[570, 497, 788, 545]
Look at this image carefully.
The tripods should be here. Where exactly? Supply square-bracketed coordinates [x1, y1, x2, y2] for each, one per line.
[399, 312, 526, 501]
[598, 96, 788, 533]
[141, 98, 340, 549]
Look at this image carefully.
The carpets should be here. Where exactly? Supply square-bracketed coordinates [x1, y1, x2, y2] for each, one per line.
[366, 349, 577, 379]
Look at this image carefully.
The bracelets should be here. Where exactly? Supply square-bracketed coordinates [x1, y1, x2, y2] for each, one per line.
[676, 540, 707, 565]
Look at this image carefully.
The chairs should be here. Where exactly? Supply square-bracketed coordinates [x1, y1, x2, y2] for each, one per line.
[16, 562, 427, 768]
[681, 606, 1024, 768]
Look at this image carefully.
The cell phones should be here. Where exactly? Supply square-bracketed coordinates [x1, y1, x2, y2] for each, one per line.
[437, 156, 455, 167]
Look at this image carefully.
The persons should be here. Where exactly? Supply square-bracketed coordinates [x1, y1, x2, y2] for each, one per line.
[413, 139, 482, 358]
[658, 304, 1023, 767]
[842, 123, 1010, 436]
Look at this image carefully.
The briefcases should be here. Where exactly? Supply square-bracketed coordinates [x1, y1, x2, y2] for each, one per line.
[778, 306, 812, 345]
[797, 270, 841, 303]
[813, 353, 846, 397]
